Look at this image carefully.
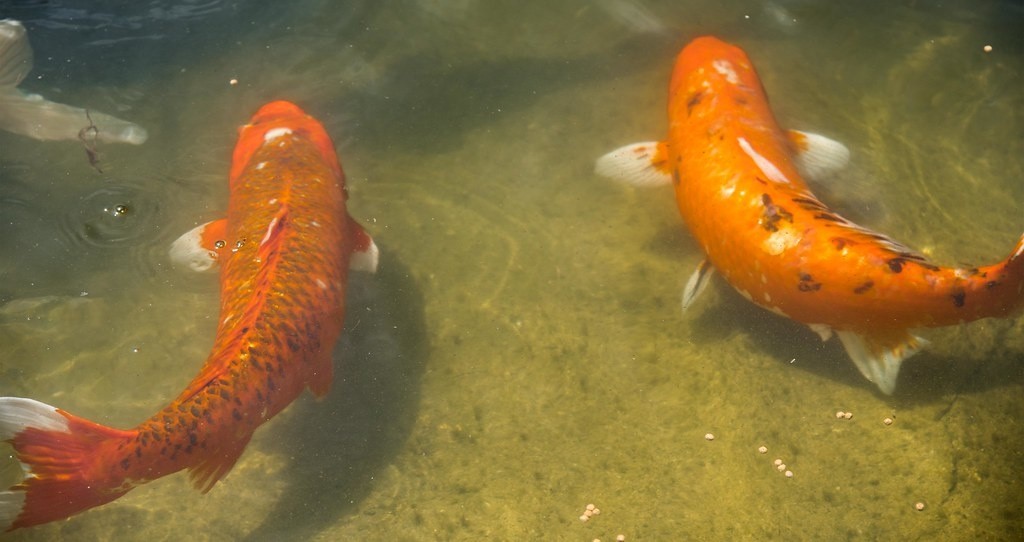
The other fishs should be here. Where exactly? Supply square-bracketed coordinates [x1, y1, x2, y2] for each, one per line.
[590, 31, 1023, 404]
[0, 95, 383, 541]
